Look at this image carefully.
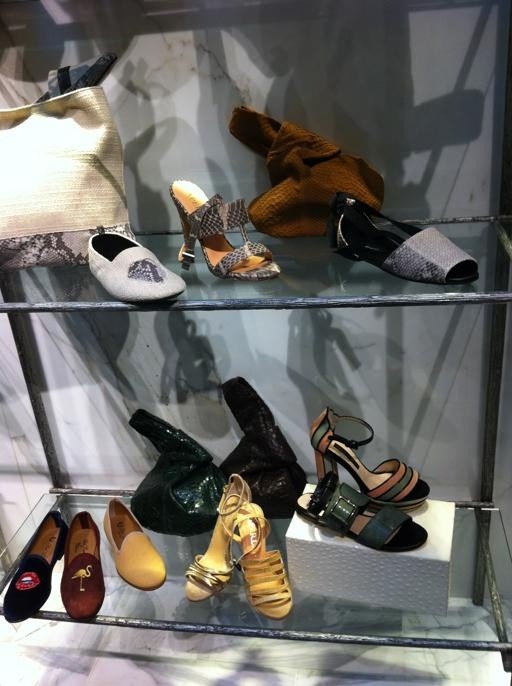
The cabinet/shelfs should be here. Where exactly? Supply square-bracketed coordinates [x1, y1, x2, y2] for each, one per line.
[0, 216, 512, 650]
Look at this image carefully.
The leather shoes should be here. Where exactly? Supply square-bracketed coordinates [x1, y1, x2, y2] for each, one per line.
[88, 226, 186, 304]
[104, 497, 166, 591]
[3, 511, 64, 622]
[61, 511, 104, 620]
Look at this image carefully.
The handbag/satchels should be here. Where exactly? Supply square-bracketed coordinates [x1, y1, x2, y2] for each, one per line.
[1, 52, 133, 269]
[130, 377, 306, 536]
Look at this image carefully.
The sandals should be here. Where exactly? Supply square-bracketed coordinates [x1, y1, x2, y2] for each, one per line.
[185, 473, 293, 620]
[330, 191, 480, 284]
[296, 474, 428, 552]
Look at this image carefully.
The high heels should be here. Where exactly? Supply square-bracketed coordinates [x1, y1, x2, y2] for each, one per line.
[169, 180, 282, 280]
[311, 406, 430, 510]
